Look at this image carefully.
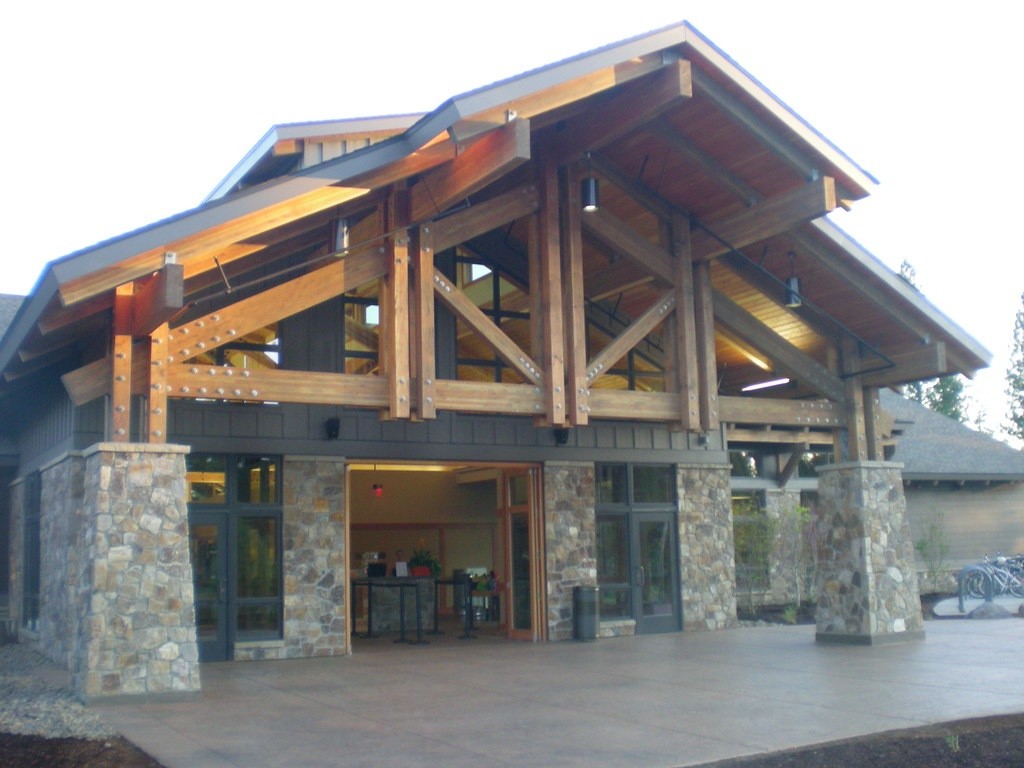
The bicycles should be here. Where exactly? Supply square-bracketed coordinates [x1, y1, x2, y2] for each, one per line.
[966, 552, 1024, 599]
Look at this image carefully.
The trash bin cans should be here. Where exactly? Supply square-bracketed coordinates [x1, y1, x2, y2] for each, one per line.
[573, 586, 601, 642]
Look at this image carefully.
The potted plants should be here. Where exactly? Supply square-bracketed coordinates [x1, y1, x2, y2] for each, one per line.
[408, 548, 441, 580]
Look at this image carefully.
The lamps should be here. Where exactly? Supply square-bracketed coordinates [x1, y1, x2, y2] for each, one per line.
[785, 256, 804, 309]
[328, 206, 350, 256]
[581, 153, 599, 213]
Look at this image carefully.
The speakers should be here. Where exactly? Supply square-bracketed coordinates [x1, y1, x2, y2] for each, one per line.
[327, 417, 340, 439]
[553, 427, 569, 444]
